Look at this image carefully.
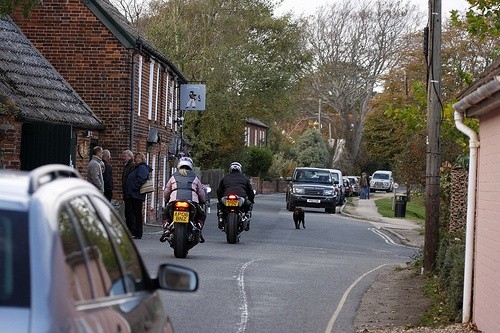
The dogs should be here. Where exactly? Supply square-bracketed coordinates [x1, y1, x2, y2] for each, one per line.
[292, 207, 305, 229]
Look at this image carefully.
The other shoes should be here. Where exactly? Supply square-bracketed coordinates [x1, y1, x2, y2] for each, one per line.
[133, 236, 140, 239]
[160, 230, 171, 242]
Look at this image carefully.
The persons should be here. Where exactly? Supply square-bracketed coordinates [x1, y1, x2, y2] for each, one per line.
[121, 149, 139, 237]
[359, 172, 370, 200]
[159, 157, 208, 244]
[123, 153, 154, 239]
[216, 162, 255, 232]
[318, 176, 328, 182]
[100, 149, 114, 202]
[87, 146, 105, 196]
[296, 171, 305, 181]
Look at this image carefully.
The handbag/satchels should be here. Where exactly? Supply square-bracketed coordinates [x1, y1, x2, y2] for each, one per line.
[140, 180, 153, 194]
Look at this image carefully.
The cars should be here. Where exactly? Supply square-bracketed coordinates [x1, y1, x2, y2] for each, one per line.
[349, 176, 360, 195]
[343, 178, 351, 197]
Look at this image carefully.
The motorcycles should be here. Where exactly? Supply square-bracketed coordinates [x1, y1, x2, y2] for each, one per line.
[162, 186, 212, 258]
[217, 190, 257, 244]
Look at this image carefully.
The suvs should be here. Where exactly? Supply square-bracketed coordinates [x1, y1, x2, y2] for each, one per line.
[314, 169, 345, 203]
[369, 171, 393, 193]
[285, 167, 339, 214]
[0, 163, 199, 333]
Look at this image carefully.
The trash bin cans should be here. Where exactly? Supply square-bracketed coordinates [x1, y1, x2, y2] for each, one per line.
[394, 194, 408, 219]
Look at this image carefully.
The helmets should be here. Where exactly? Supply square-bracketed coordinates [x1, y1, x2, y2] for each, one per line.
[229, 162, 242, 173]
[176, 156, 193, 170]
